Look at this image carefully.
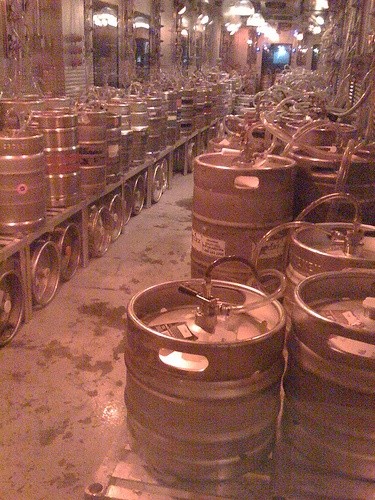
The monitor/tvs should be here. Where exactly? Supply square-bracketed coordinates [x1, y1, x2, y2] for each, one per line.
[271, 43, 292, 66]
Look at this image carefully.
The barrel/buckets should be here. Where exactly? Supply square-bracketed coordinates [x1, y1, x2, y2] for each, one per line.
[282, 269, 375, 485]
[124, 280, 287, 487]
[0, 68, 374, 347]
